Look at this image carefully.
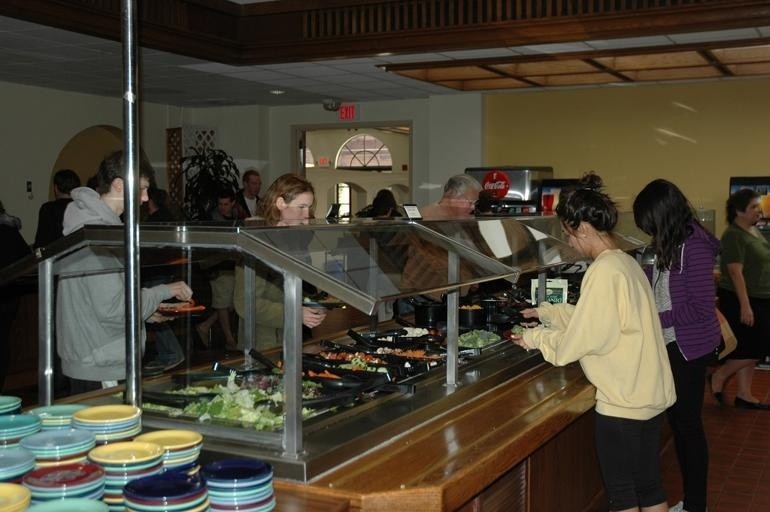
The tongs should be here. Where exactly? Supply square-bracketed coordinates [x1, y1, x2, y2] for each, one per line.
[492, 313, 540, 328]
[316, 373, 416, 400]
[440, 348, 482, 359]
[320, 329, 381, 355]
[170, 349, 279, 383]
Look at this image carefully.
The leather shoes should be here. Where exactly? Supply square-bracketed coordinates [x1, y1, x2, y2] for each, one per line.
[708, 373, 724, 403]
[734, 396, 769, 409]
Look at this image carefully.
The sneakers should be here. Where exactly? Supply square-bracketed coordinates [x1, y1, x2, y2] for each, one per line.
[225, 343, 241, 353]
[161, 354, 186, 371]
[143, 360, 165, 369]
[196, 321, 211, 348]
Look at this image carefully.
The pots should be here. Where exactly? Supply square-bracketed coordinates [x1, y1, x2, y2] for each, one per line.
[407, 292, 504, 329]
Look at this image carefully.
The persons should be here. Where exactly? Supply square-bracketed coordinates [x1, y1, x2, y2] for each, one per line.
[55, 151, 193, 396]
[399, 173, 484, 315]
[194, 188, 245, 350]
[233, 174, 327, 355]
[232, 170, 262, 220]
[633, 177, 726, 512]
[355, 189, 410, 321]
[140, 188, 185, 374]
[703, 188, 770, 411]
[505, 169, 680, 512]
[87, 174, 104, 193]
[0, 208, 33, 379]
[34, 169, 81, 252]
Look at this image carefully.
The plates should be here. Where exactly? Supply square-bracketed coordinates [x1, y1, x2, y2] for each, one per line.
[1, 395, 278, 511]
[503, 328, 526, 340]
[157, 305, 207, 318]
[301, 298, 345, 310]
